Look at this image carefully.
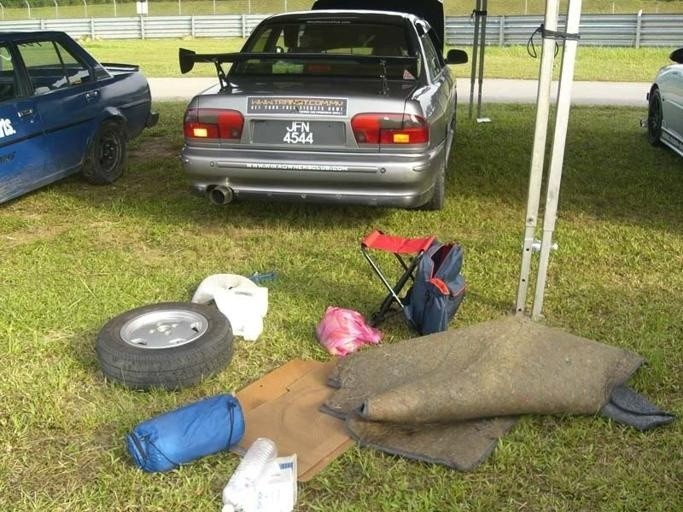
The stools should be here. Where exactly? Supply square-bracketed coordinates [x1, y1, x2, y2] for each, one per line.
[361, 230, 435, 335]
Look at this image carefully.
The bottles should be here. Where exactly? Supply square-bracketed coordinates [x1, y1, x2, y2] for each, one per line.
[211, 437, 278, 512]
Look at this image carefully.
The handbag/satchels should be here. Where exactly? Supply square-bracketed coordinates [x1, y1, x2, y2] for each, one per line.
[405, 242, 467, 335]
[130, 394, 245, 473]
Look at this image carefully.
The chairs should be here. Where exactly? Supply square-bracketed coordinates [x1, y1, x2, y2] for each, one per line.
[288, 34, 322, 54]
[371, 43, 406, 56]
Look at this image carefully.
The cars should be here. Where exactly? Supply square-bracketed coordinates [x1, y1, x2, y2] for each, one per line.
[178, 1, 470, 213]
[0, 31, 161, 209]
[640, 47, 683, 159]
